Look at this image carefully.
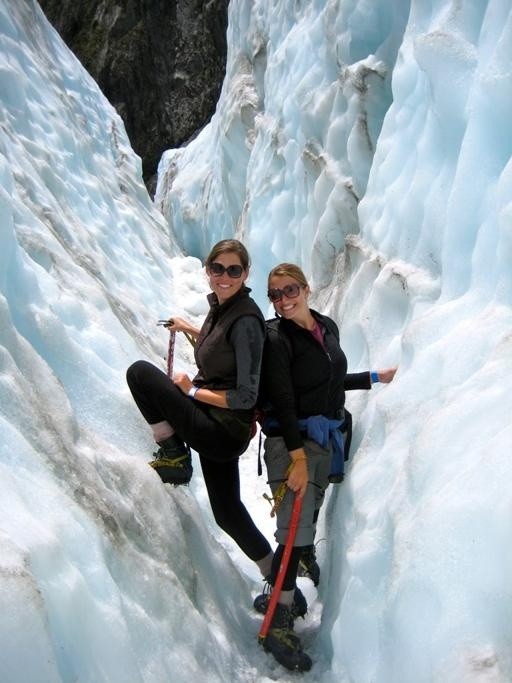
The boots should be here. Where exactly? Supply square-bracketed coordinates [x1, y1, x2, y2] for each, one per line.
[255, 549, 321, 671]
[147, 435, 191, 483]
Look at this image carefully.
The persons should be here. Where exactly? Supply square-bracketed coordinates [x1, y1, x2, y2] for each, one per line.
[125, 237, 308, 619]
[255, 263, 399, 674]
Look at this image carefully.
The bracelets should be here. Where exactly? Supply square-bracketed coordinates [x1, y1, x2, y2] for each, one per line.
[189, 384, 201, 398]
[370, 369, 379, 383]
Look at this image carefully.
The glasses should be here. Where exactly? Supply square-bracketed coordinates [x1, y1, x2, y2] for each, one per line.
[269, 284, 304, 302]
[208, 264, 246, 278]
